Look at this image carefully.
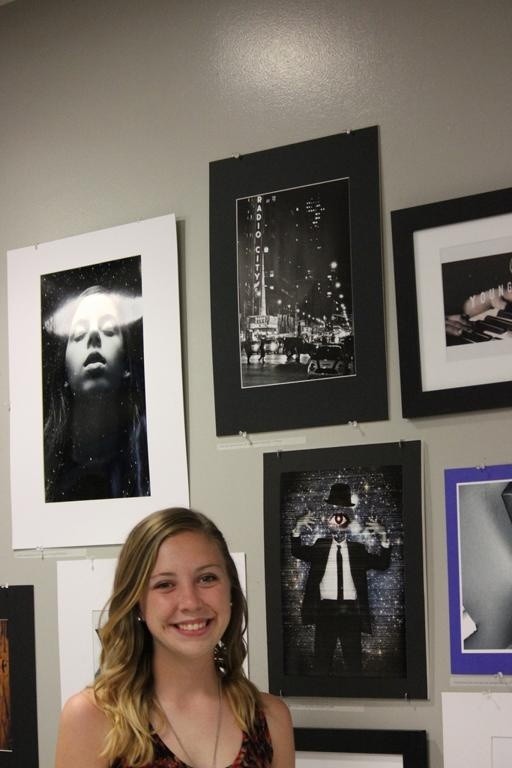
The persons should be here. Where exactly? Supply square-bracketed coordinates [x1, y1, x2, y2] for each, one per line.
[40, 284, 151, 501]
[244, 337, 253, 364]
[52, 505, 298, 768]
[285, 508, 394, 674]
[257, 340, 266, 364]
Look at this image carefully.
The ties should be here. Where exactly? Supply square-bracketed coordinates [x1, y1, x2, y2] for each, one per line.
[336, 544, 344, 603]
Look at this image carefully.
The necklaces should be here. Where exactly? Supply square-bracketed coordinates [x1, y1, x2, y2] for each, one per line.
[152, 671, 223, 768]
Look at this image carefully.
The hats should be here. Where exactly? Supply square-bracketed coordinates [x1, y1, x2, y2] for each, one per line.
[323, 483, 355, 507]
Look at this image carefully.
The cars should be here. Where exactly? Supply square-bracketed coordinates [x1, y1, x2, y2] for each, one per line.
[246, 329, 355, 379]
